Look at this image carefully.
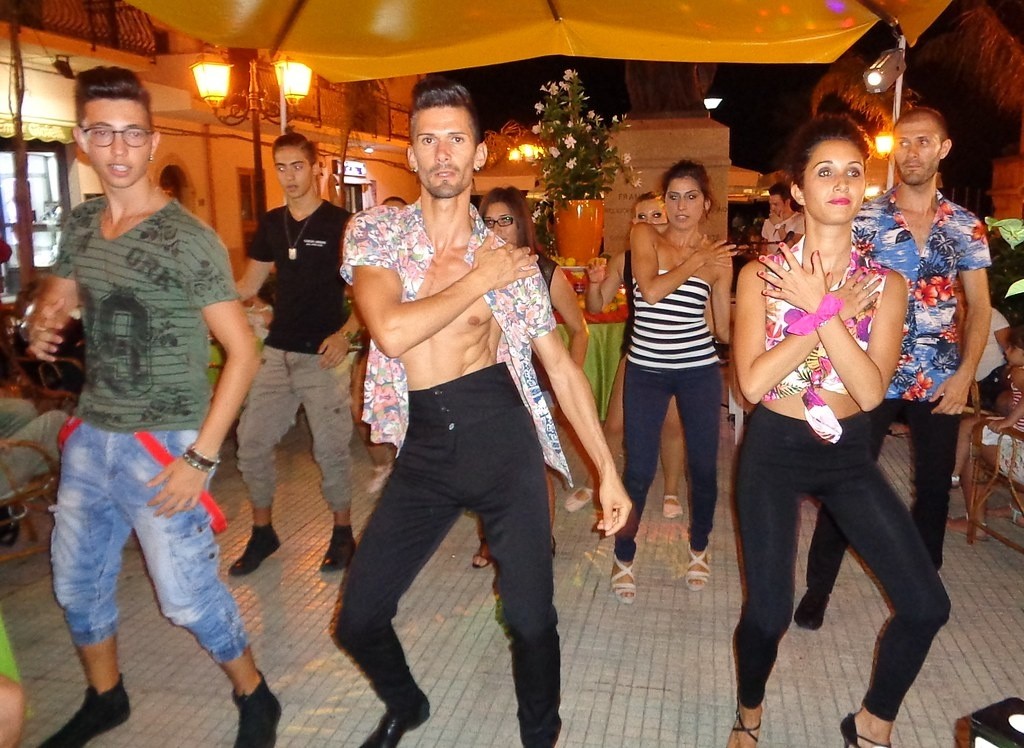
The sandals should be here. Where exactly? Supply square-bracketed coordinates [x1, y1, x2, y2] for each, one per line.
[472, 539, 492, 567]
[611, 553, 637, 604]
[685, 542, 710, 590]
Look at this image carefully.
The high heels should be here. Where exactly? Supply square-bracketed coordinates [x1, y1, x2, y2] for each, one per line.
[726, 705, 762, 742]
[840, 713, 893, 748]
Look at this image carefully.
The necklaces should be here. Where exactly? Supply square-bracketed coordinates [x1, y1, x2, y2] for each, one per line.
[284, 202, 318, 260]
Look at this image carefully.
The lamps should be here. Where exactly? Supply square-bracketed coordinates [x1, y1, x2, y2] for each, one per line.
[53, 53, 77, 79]
[863, 49, 907, 94]
[192, 50, 312, 224]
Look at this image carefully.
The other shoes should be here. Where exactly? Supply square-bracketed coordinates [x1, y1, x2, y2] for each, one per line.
[232, 670, 281, 748]
[566, 486, 594, 511]
[37, 673, 131, 748]
[795, 588, 829, 629]
[663, 495, 683, 518]
[321, 523, 356, 571]
[230, 524, 282, 576]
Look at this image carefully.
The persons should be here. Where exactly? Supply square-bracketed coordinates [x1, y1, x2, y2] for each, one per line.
[229, 135, 363, 578]
[0, 398, 75, 547]
[761, 180, 806, 257]
[21, 66, 281, 748]
[609, 160, 737, 605]
[725, 115, 950, 748]
[730, 216, 748, 242]
[0, 605, 37, 748]
[331, 74, 633, 748]
[563, 192, 685, 518]
[946, 271, 1024, 541]
[793, 107, 991, 629]
[474, 186, 590, 568]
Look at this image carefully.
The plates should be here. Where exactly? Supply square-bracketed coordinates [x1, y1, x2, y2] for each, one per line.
[559, 266, 587, 271]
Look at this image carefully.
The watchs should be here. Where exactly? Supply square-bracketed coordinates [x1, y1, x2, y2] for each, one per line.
[774, 222, 785, 229]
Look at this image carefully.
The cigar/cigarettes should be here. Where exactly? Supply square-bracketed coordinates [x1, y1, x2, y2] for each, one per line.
[768, 214, 772, 216]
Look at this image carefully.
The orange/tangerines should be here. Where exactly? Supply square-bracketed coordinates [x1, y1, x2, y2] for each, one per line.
[550, 256, 577, 266]
[577, 291, 627, 313]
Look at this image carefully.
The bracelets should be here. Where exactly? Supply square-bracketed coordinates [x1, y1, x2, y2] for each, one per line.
[338, 327, 354, 341]
[338, 328, 355, 340]
[183, 448, 221, 473]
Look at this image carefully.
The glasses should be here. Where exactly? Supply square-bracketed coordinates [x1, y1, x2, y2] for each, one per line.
[484, 216, 516, 230]
[77, 123, 153, 147]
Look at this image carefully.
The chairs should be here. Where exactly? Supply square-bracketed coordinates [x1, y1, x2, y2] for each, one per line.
[0, 304, 88, 564]
[962, 418, 1024, 553]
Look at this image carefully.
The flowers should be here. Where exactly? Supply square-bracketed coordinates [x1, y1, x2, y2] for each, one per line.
[522, 70, 646, 229]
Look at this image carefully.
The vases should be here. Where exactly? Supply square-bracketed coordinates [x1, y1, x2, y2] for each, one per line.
[553, 200, 604, 266]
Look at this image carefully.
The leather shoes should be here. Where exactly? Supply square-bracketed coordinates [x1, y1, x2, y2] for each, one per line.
[361, 698, 431, 748]
